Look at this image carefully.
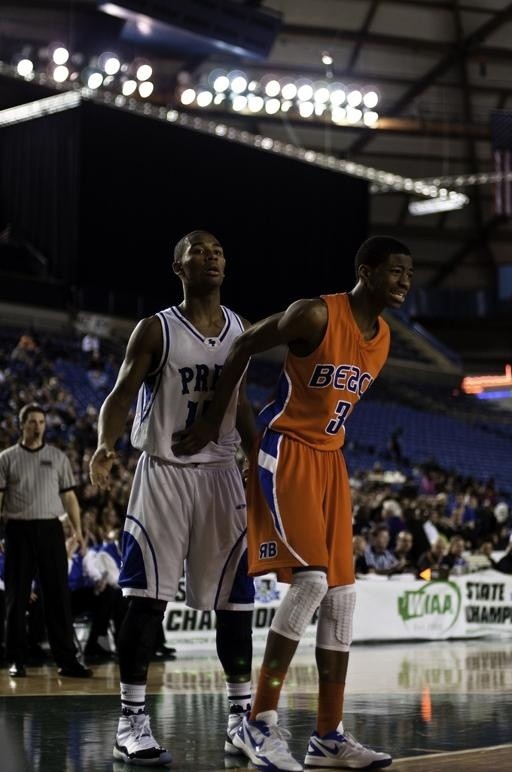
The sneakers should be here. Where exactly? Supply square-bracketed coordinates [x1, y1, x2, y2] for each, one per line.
[112, 708, 174, 766]
[304, 720, 393, 770]
[223, 703, 304, 771]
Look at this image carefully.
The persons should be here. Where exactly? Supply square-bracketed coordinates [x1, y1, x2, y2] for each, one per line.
[89, 229, 259, 767]
[172, 235, 414, 772]
[1, 326, 511, 679]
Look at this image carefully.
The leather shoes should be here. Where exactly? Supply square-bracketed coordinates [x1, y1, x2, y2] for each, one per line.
[152, 645, 175, 656]
[9, 663, 26, 677]
[84, 644, 117, 658]
[58, 658, 93, 678]
